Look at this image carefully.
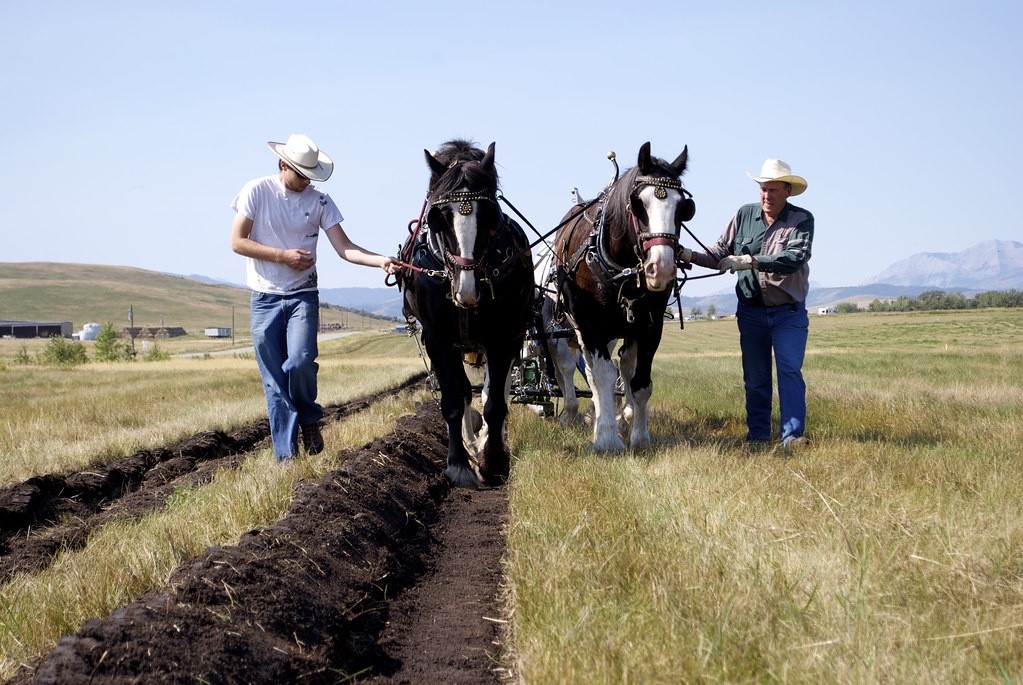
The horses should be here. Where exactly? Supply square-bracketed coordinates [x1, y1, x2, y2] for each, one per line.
[540, 141, 696, 456]
[397, 139, 536, 488]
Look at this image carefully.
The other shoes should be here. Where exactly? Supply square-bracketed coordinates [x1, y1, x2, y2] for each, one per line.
[785, 437, 809, 456]
[749, 441, 770, 452]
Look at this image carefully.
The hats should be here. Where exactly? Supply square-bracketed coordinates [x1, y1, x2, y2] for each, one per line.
[266, 134, 334, 182]
[753, 160, 807, 196]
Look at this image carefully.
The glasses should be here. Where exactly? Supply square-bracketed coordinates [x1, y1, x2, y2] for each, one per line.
[287, 165, 306, 179]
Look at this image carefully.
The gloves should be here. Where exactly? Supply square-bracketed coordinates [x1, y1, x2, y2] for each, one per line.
[718, 254, 752, 274]
[678, 248, 692, 262]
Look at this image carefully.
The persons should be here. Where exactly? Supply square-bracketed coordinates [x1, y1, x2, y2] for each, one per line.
[677, 158, 814, 455]
[230, 134, 402, 464]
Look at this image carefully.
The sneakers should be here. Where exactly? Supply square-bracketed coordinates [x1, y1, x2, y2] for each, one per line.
[299, 422, 324, 455]
